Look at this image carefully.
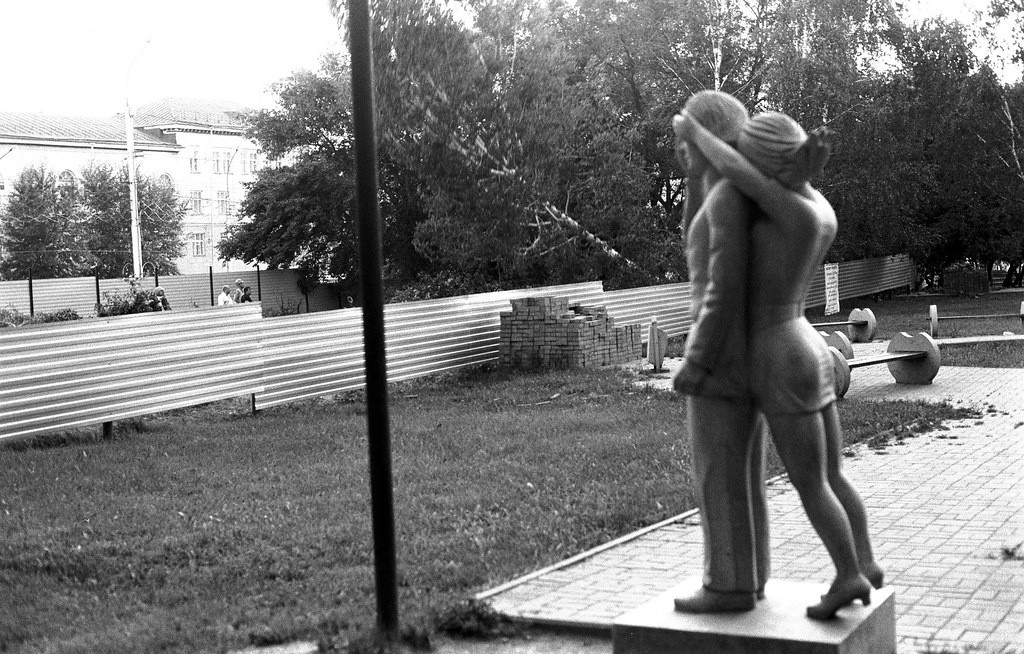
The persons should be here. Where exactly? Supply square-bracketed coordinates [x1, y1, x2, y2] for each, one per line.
[671, 88, 884, 621]
[233, 278, 253, 304]
[153, 286, 172, 311]
[218, 285, 233, 306]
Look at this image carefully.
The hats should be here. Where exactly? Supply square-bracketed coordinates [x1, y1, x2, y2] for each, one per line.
[235, 279, 245, 286]
[154, 286, 164, 295]
